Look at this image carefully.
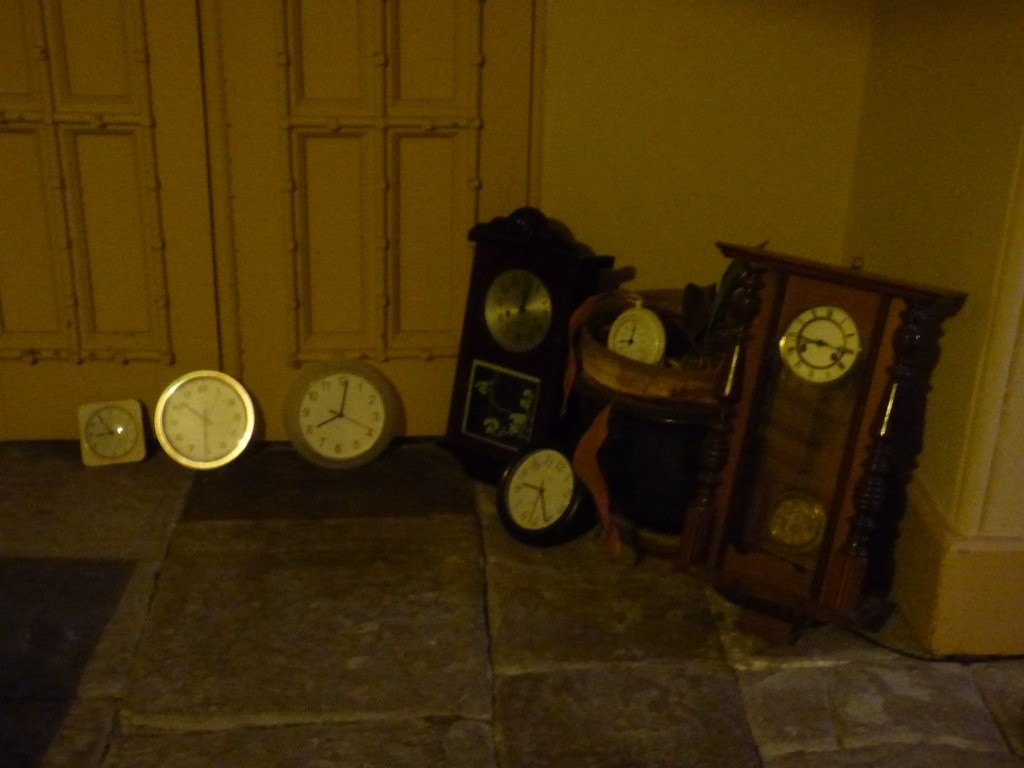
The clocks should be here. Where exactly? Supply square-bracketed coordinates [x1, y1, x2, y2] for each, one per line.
[679, 239, 967, 648]
[77, 398, 146, 468]
[495, 445, 588, 547]
[441, 205, 616, 483]
[154, 369, 256, 470]
[282, 358, 400, 470]
[554, 281, 738, 569]
